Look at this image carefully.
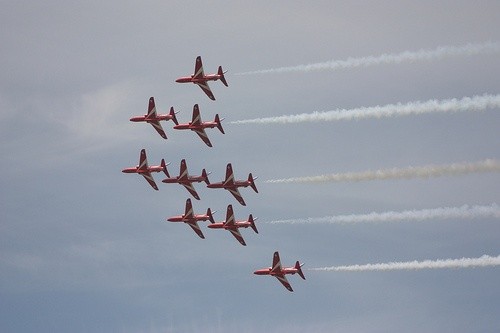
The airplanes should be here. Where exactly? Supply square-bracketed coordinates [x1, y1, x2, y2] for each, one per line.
[173, 103, 226, 148]
[206, 162, 259, 206]
[121, 148, 171, 191]
[161, 158, 211, 201]
[166, 197, 215, 240]
[175, 55, 229, 101]
[129, 96, 180, 140]
[252, 251, 306, 293]
[207, 204, 260, 247]
